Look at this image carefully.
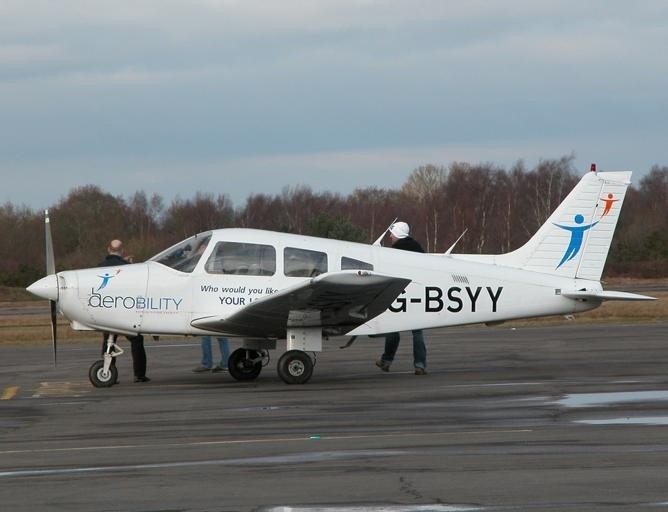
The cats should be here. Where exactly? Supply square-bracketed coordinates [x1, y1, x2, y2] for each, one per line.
[26, 162, 661, 389]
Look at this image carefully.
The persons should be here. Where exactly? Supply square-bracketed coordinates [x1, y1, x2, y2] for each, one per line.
[192, 243, 230, 374]
[376, 221, 427, 375]
[94, 239, 151, 383]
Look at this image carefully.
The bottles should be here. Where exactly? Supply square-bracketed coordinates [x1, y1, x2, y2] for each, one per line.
[389, 222, 409, 239]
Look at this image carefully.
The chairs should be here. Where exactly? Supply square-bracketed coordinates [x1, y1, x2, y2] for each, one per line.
[414, 367, 427, 374]
[192, 366, 209, 371]
[213, 367, 229, 372]
[375, 358, 389, 370]
[133, 375, 150, 383]
